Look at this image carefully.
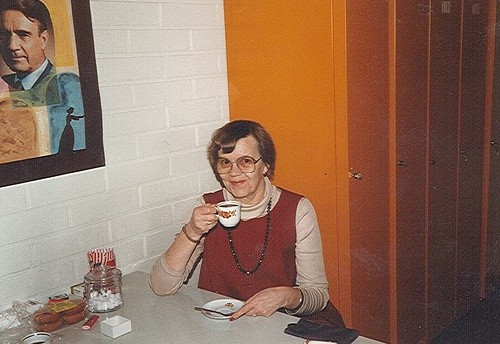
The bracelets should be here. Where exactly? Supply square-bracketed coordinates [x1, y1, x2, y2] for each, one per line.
[287, 288, 304, 311]
[182, 226, 200, 244]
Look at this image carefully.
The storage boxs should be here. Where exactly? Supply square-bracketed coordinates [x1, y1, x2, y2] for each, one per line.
[100, 315, 132, 340]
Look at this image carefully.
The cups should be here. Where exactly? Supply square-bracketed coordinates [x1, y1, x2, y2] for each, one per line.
[21, 332, 52, 344]
[215, 200, 241, 227]
[84, 266, 124, 313]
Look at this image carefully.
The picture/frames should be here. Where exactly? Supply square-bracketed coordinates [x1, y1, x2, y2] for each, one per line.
[0, 0, 106, 189]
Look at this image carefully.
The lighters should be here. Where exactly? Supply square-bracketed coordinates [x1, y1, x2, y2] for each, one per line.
[82, 315, 99, 330]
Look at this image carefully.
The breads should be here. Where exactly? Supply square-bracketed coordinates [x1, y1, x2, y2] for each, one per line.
[33, 299, 89, 332]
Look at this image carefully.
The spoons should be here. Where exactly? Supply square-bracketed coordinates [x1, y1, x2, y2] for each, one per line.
[195, 307, 235, 316]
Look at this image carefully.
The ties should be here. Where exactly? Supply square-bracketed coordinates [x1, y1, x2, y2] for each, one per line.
[15, 81, 22, 90]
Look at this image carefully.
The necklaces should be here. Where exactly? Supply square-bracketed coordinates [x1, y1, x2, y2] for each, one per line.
[227, 199, 271, 274]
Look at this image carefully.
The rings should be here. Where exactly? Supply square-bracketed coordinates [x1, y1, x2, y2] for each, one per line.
[253, 314, 256, 316]
[208, 221, 211, 225]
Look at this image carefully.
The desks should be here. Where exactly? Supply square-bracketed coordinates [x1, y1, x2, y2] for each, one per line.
[0, 270, 386, 344]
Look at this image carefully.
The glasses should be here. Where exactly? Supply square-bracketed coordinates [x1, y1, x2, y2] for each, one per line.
[213, 155, 261, 174]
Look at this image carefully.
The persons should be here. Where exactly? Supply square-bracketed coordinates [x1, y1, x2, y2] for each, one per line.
[148, 120, 346, 327]
[0, 0, 53, 89]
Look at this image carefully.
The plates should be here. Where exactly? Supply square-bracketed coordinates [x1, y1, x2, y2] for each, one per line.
[202, 298, 243, 319]
